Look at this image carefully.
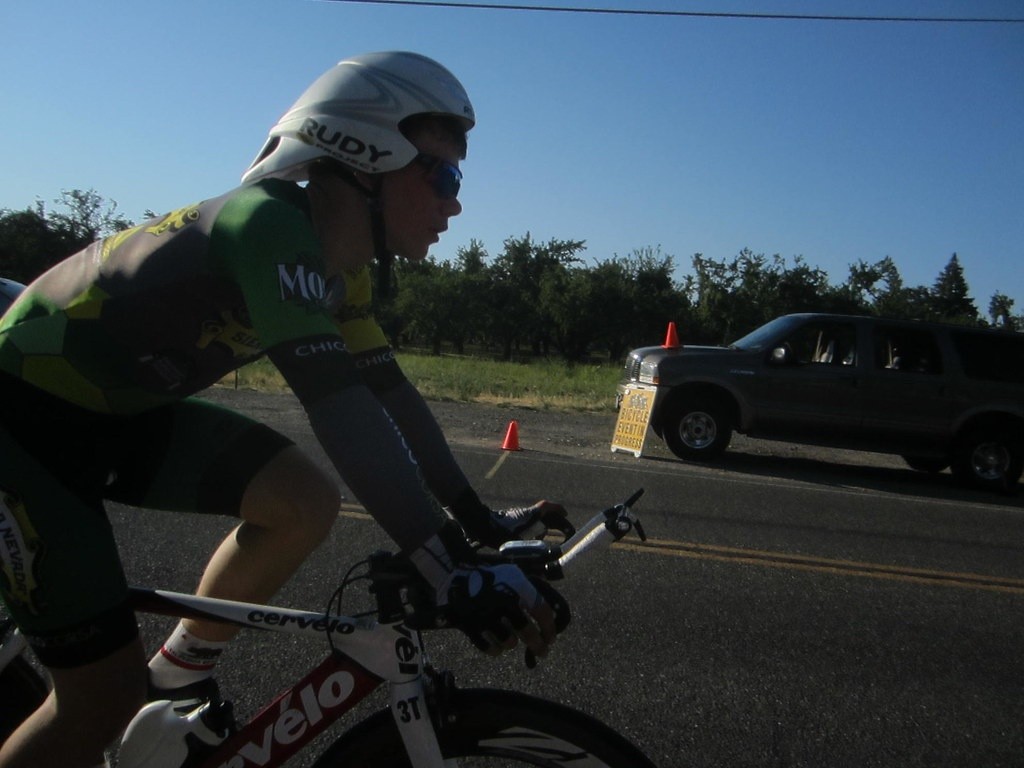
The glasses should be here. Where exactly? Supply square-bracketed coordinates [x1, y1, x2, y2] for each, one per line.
[416, 152, 463, 205]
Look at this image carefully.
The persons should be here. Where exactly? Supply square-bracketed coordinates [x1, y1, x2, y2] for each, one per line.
[0, 51, 569, 768]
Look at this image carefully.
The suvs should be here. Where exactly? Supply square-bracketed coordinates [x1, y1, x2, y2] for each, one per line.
[612, 313, 1023, 498]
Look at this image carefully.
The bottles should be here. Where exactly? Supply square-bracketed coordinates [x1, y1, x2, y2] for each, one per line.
[120, 699, 235, 768]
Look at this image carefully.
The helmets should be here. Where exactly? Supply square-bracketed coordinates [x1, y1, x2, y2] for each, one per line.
[0, 278, 27, 318]
[240, 51, 474, 185]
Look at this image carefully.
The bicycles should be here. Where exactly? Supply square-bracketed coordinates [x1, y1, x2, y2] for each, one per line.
[1, 487, 661, 768]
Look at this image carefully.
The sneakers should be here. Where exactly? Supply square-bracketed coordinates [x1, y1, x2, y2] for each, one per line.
[138, 653, 238, 768]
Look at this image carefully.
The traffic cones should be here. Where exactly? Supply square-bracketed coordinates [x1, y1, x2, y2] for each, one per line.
[500, 421, 524, 451]
[663, 321, 683, 350]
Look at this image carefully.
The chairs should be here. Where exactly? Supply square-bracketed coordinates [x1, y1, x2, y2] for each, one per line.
[821, 337, 856, 365]
